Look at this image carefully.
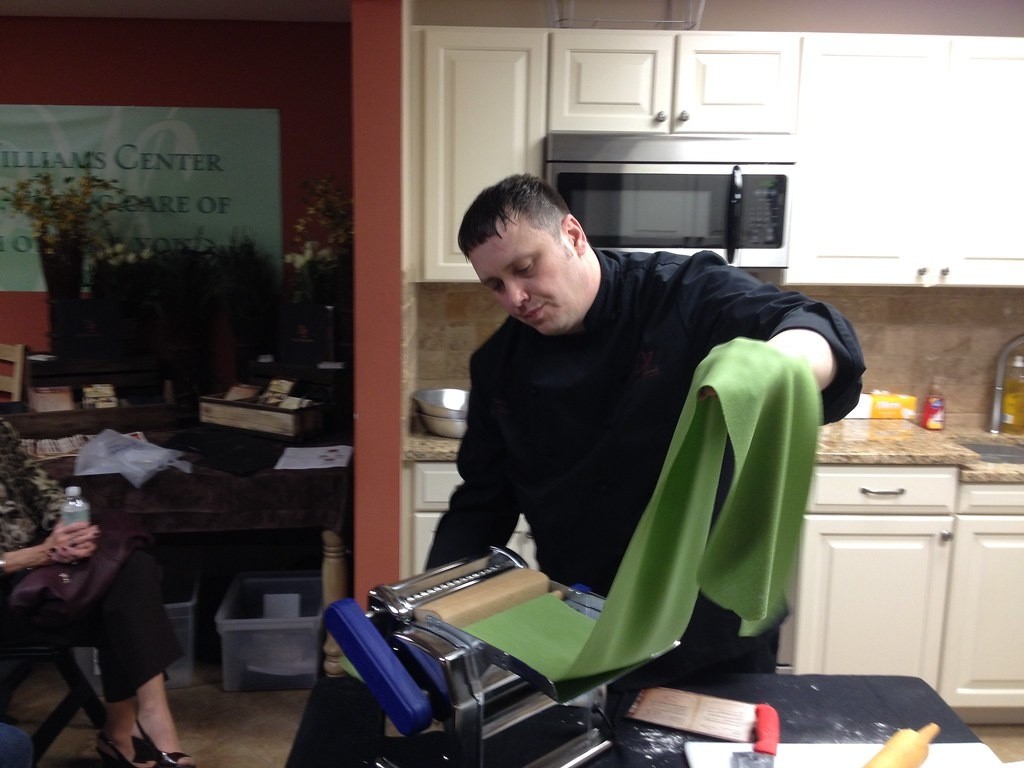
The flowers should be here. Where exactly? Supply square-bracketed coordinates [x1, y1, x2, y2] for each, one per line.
[92, 239, 153, 266]
[0, 166, 133, 253]
[289, 177, 352, 265]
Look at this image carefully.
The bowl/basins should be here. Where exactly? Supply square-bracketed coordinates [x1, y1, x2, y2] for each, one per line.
[412, 410, 467, 438]
[411, 388, 470, 419]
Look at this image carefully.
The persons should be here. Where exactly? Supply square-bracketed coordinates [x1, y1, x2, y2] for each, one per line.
[422, 174, 865, 674]
[0, 413, 198, 768]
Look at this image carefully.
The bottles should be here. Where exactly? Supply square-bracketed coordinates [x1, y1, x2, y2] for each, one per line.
[60, 486, 90, 566]
[921, 382, 945, 430]
[1000, 355, 1024, 434]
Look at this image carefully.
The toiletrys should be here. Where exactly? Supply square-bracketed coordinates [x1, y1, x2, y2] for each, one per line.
[1001, 354, 1024, 435]
[922, 376, 945, 430]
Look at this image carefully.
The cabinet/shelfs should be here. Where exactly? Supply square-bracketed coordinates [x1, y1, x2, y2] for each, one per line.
[404, 457, 1024, 726]
[402, 24, 1022, 287]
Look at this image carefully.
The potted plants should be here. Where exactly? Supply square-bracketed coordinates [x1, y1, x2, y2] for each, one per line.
[185, 240, 257, 384]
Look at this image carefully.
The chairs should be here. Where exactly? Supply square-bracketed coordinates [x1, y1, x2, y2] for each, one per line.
[0, 569, 105, 768]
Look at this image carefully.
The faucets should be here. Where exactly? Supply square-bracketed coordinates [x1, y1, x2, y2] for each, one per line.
[989, 334, 1024, 434]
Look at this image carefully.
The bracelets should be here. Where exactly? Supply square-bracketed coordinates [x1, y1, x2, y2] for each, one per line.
[70, 540, 76, 546]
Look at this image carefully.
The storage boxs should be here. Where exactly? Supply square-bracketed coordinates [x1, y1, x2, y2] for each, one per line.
[196, 393, 326, 441]
[70, 568, 204, 698]
[212, 569, 327, 694]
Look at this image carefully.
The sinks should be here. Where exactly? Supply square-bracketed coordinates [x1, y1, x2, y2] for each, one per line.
[959, 443, 1024, 464]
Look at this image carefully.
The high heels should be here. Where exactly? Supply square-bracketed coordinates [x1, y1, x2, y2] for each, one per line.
[96, 730, 160, 768]
[130, 720, 195, 768]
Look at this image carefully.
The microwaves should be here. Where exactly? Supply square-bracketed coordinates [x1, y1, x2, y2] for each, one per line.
[544, 130, 801, 270]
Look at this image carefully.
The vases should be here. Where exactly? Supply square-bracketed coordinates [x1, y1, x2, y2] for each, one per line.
[310, 262, 354, 305]
[97, 263, 146, 300]
[38, 241, 82, 299]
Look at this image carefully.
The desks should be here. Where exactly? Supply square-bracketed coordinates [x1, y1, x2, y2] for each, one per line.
[30, 427, 353, 604]
[286, 673, 983, 766]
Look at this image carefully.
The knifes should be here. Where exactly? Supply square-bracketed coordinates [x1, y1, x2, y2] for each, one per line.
[732, 703, 780, 767]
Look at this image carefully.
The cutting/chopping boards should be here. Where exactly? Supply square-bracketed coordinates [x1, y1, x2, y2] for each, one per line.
[683, 742, 1003, 768]
